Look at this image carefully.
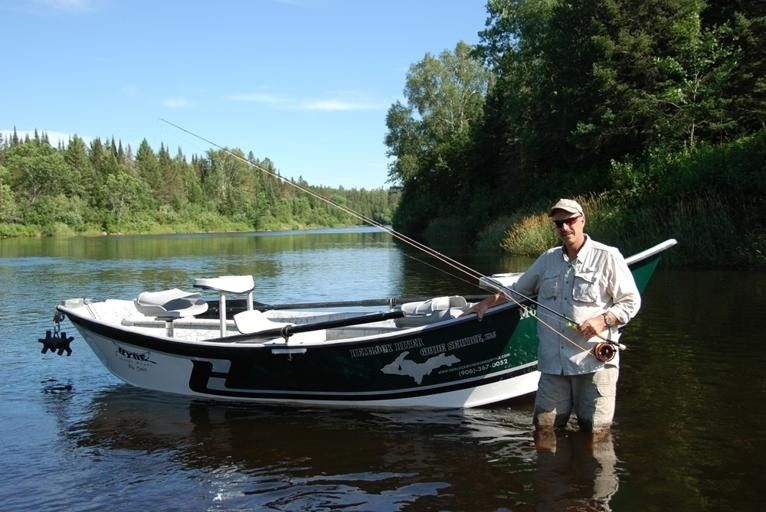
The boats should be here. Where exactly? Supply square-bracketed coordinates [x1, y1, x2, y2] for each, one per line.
[60, 235, 677, 414]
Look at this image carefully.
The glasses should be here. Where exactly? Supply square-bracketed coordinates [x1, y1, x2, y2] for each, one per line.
[553, 215, 582, 228]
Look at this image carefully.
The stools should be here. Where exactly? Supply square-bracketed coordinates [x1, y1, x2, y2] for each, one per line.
[136, 273, 298, 344]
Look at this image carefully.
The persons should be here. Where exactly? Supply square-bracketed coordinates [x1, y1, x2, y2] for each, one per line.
[459, 197, 645, 431]
[529, 430, 619, 512]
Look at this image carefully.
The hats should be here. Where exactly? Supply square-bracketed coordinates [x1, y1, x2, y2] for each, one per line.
[548, 198, 585, 217]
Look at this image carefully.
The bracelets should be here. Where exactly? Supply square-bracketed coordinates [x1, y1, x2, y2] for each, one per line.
[602, 312, 612, 328]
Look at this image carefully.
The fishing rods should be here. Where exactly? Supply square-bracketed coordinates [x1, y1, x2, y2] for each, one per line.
[160, 118, 631, 361]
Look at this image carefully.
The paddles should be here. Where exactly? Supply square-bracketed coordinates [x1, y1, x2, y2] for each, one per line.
[201, 295, 450, 343]
[192, 294, 494, 320]
